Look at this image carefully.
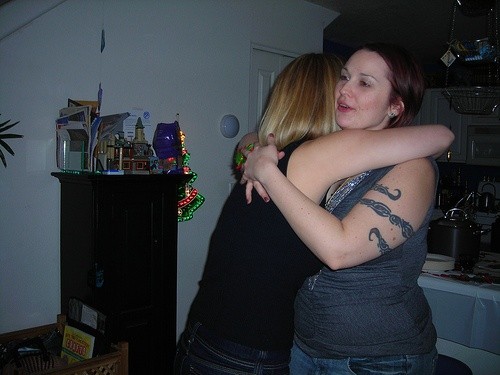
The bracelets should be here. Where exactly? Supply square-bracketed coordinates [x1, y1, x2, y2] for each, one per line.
[233, 141, 257, 171]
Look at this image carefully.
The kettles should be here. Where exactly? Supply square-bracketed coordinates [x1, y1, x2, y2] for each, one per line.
[475, 184, 497, 212]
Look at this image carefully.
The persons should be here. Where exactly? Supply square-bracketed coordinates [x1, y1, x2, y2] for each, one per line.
[230, 39, 447, 375]
[169, 51, 455, 375]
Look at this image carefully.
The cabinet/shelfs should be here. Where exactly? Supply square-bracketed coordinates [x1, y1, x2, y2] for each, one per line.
[51, 171, 193, 375]
[408, 85, 500, 166]
